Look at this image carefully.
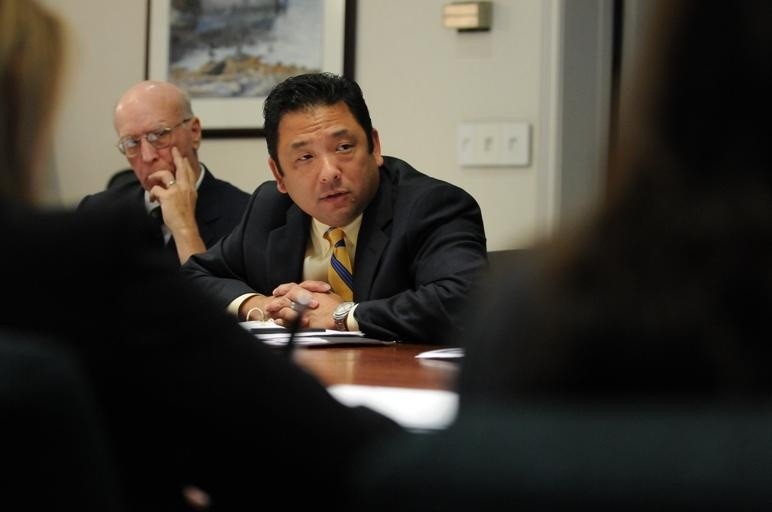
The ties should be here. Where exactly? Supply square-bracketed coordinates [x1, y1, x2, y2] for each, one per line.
[325, 226, 353, 303]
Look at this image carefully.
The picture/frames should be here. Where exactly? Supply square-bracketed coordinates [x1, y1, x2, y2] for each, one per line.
[144, 0, 356, 138]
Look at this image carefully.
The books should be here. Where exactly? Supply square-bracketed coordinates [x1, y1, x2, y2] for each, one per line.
[238, 317, 395, 345]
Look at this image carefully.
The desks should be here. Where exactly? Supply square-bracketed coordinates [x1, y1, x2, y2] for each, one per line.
[273, 343, 465, 396]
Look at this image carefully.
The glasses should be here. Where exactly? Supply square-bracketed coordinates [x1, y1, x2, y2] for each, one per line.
[114, 120, 190, 161]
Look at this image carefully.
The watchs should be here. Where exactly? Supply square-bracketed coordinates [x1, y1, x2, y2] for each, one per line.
[331, 301, 355, 331]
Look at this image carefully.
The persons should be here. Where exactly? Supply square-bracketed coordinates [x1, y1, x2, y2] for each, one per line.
[1, 0, 411, 511]
[409, 1, 772, 511]
[174, 72, 490, 345]
[73, 79, 251, 267]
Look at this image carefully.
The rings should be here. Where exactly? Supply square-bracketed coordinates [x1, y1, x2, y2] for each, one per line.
[167, 179, 176, 188]
[290, 301, 296, 309]
